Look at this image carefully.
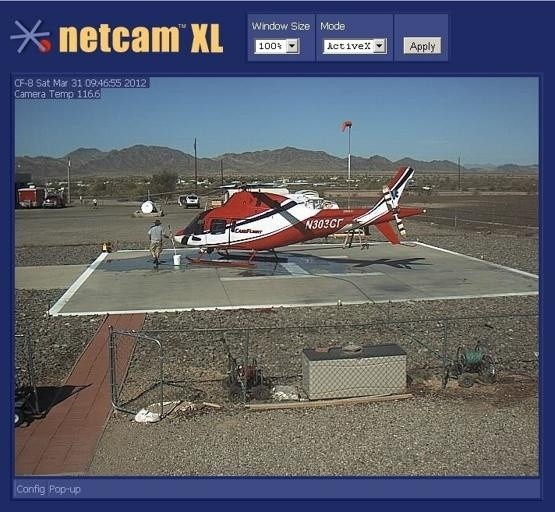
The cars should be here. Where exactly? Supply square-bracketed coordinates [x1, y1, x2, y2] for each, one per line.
[178, 194, 199, 209]
[42, 195, 65, 208]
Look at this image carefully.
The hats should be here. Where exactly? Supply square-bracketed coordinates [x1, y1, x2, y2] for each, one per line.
[153, 220, 162, 224]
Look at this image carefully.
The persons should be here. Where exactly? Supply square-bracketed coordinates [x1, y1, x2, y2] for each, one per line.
[148, 219, 171, 265]
[93, 197, 98, 209]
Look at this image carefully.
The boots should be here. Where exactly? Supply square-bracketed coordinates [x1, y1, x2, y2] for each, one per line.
[152, 258, 158, 265]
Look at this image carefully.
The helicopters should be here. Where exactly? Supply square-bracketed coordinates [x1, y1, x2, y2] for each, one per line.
[120, 166, 426, 266]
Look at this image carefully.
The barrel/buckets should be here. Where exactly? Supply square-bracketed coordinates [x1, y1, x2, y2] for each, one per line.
[173, 255, 182, 265]
